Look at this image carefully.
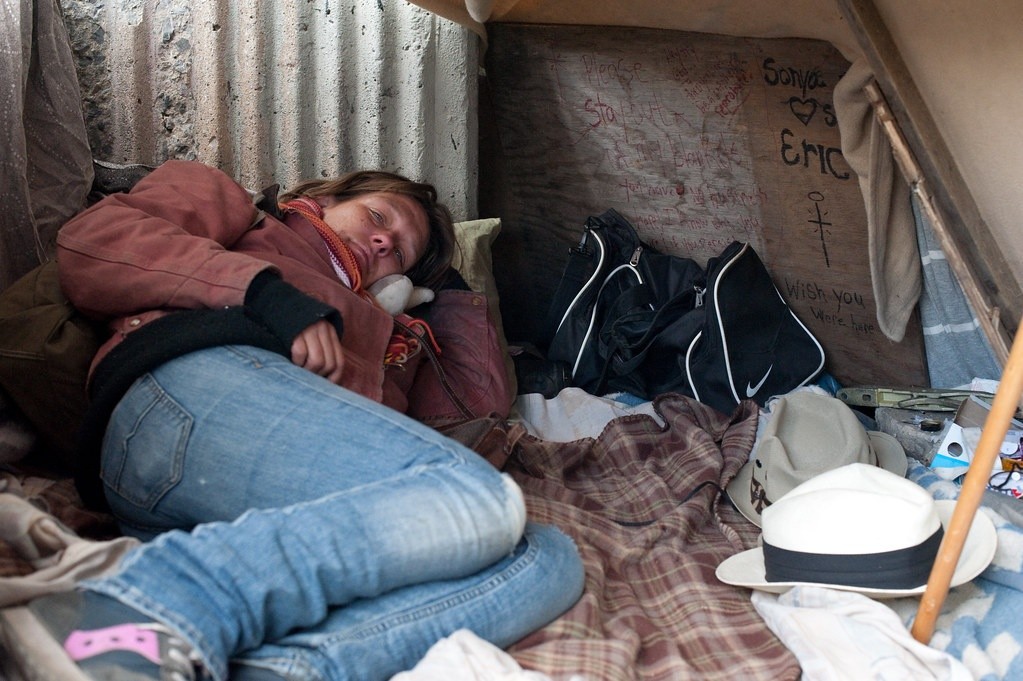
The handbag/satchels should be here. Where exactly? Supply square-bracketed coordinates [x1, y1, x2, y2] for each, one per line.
[525, 206, 827, 418]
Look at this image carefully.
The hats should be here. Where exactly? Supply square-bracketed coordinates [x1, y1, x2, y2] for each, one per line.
[714, 462, 998, 598]
[723, 387, 909, 529]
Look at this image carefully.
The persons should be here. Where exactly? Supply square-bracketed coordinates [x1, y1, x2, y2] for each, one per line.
[0, 170, 586, 681]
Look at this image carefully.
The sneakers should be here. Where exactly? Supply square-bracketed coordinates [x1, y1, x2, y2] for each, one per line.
[0, 589, 292, 681]
